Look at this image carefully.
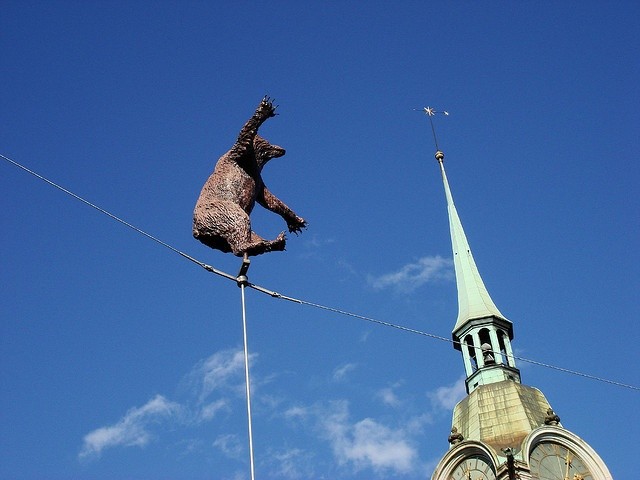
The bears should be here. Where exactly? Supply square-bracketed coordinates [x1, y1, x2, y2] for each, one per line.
[192, 94, 309, 257]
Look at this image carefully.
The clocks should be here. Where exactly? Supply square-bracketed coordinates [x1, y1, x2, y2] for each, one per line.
[430, 440, 501, 480]
[520, 425, 614, 480]
